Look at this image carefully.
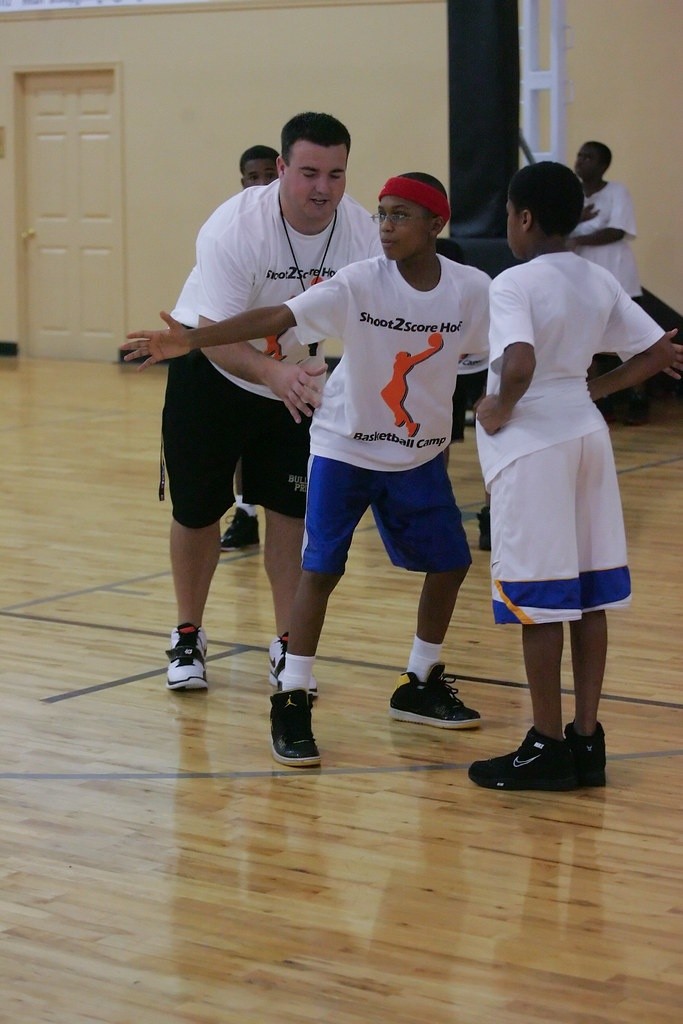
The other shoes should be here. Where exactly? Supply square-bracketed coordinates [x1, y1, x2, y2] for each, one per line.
[624, 392, 649, 425]
[596, 398, 617, 422]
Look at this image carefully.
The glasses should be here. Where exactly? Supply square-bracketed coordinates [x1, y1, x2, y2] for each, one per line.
[370, 211, 436, 225]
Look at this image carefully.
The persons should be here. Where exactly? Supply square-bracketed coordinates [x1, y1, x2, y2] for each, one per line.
[115, 110, 683, 794]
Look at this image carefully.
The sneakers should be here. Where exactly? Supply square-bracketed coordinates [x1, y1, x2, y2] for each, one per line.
[564, 719, 606, 784]
[467, 726, 577, 791]
[269, 631, 318, 696]
[220, 508, 260, 550]
[387, 662, 483, 729]
[477, 506, 492, 551]
[269, 688, 321, 766]
[166, 623, 208, 690]
